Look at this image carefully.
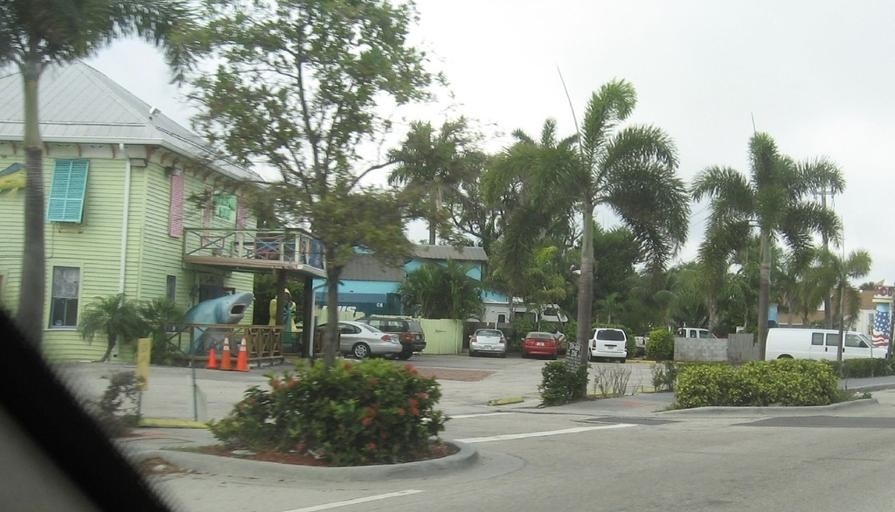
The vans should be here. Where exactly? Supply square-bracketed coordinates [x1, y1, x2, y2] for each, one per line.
[588, 328, 628, 363]
[765, 328, 893, 361]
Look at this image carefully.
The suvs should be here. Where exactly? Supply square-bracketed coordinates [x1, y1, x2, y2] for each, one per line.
[356, 316, 426, 360]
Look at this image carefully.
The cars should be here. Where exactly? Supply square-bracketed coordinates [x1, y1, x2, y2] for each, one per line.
[468, 329, 507, 358]
[317, 321, 403, 360]
[521, 332, 558, 360]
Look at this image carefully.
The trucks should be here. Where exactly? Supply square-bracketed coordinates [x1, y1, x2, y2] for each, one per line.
[634, 328, 717, 348]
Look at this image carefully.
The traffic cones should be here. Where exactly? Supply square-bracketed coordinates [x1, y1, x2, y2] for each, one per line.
[205, 348, 218, 369]
[219, 337, 233, 370]
[233, 337, 250, 371]
